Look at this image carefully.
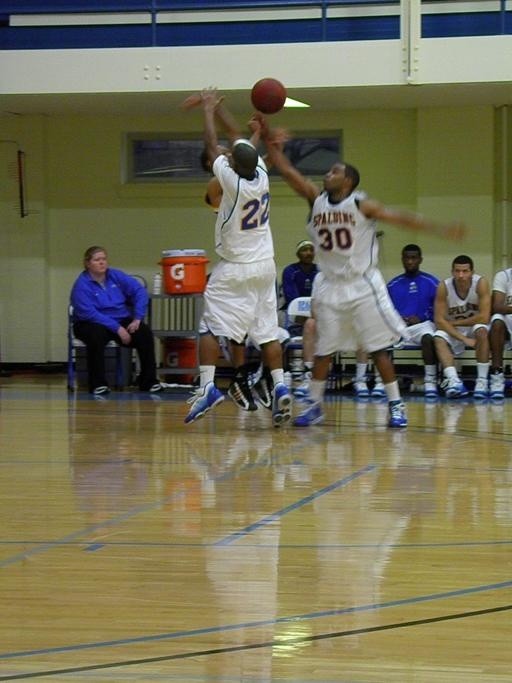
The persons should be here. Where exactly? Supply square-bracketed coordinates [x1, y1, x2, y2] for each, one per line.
[185, 87, 295, 431]
[201, 123, 286, 413]
[373, 244, 438, 399]
[432, 257, 492, 398]
[488, 269, 512, 402]
[70, 246, 163, 397]
[247, 115, 464, 428]
[224, 341, 274, 408]
[281, 240, 317, 304]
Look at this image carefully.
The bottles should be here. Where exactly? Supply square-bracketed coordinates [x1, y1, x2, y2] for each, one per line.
[152, 272, 161, 296]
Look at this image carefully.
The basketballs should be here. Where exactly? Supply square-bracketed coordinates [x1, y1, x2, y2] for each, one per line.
[251, 77, 287, 114]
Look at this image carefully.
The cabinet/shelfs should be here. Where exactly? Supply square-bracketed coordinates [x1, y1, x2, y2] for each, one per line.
[149, 290, 204, 390]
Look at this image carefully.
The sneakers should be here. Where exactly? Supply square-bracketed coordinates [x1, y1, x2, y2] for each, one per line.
[424, 373, 440, 397]
[293, 374, 311, 397]
[253, 374, 273, 409]
[92, 385, 109, 395]
[489, 373, 507, 398]
[227, 377, 258, 411]
[293, 396, 326, 428]
[149, 384, 162, 393]
[442, 376, 469, 397]
[183, 381, 225, 425]
[473, 378, 489, 399]
[371, 378, 387, 396]
[388, 400, 408, 427]
[271, 383, 293, 428]
[351, 376, 370, 396]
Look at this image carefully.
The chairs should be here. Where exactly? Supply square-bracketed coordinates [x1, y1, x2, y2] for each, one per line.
[67, 302, 130, 392]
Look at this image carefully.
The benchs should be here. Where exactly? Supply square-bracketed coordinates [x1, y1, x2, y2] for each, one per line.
[336, 339, 512, 394]
